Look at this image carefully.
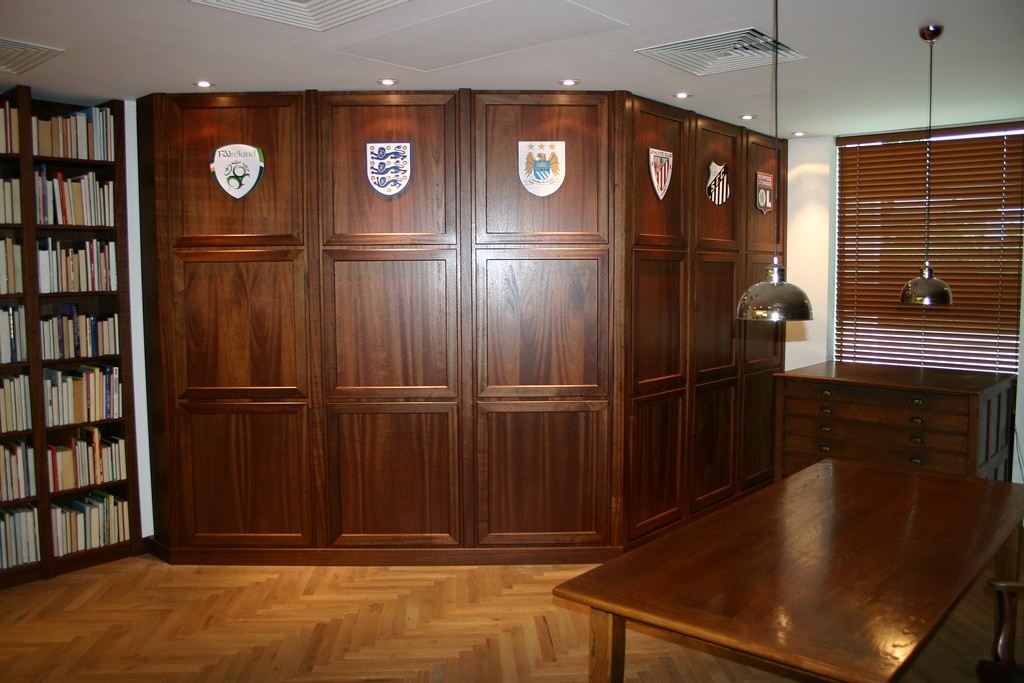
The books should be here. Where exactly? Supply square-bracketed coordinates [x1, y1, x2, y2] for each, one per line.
[0, 441, 36, 502]
[47, 426, 127, 492]
[0, 237, 23, 295]
[50, 490, 130, 557]
[34, 164, 115, 227]
[36, 235, 118, 293]
[0, 306, 27, 364]
[0, 100, 20, 154]
[0, 178, 22, 224]
[43, 363, 122, 428]
[0, 504, 41, 569]
[31, 107, 115, 161]
[0, 374, 33, 433]
[40, 303, 120, 360]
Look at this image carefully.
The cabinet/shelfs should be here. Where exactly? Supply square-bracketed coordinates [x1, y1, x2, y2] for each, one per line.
[0, 86, 140, 588]
[774, 360, 1016, 480]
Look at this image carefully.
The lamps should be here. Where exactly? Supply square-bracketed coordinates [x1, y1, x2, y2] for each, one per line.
[732, 0, 813, 323]
[899, 25, 953, 308]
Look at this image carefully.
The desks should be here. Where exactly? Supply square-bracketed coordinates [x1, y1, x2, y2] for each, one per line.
[553, 454, 1024, 683]
